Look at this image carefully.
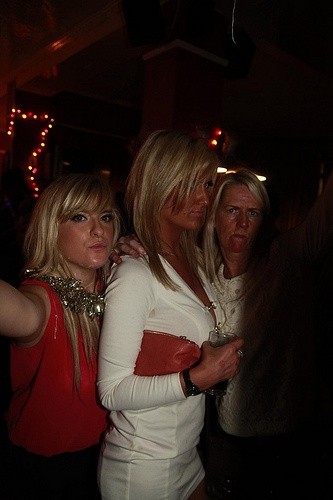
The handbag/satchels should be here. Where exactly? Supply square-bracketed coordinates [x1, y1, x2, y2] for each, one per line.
[133, 330, 203, 377]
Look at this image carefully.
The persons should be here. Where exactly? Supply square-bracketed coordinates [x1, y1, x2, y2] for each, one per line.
[200, 169, 283, 500]
[97, 129, 250, 500]
[0, 172, 147, 500]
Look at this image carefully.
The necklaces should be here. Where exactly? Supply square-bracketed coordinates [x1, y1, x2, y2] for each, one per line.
[24, 268, 107, 320]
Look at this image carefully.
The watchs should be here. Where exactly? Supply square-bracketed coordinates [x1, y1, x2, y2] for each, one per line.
[183, 367, 200, 396]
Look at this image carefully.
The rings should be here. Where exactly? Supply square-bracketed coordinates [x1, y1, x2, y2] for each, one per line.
[238, 350, 246, 359]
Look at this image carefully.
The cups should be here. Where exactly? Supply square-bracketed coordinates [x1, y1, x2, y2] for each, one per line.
[204, 331, 237, 395]
